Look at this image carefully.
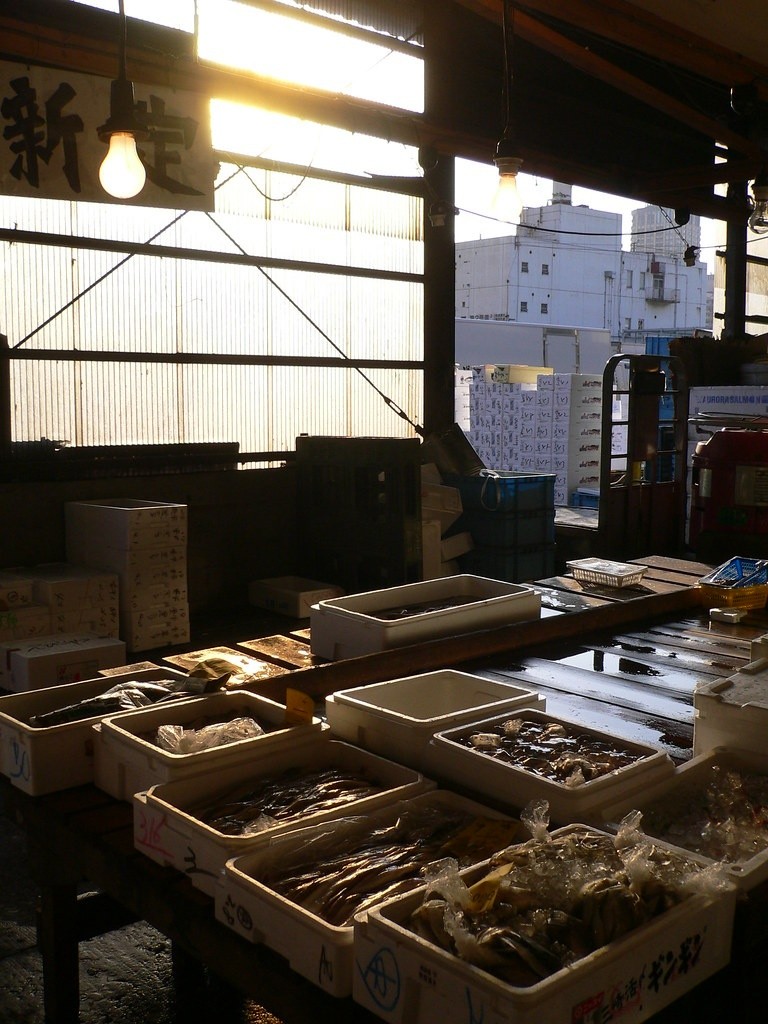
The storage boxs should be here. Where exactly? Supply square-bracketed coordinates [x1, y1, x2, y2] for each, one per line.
[692, 657, 768, 759]
[644, 336, 675, 418]
[249, 574, 347, 619]
[310, 574, 540, 661]
[325, 668, 546, 769]
[421, 364, 601, 580]
[684, 358, 768, 545]
[578, 745, 768, 894]
[612, 394, 628, 455]
[352, 823, 737, 1024]
[133, 740, 439, 900]
[91, 689, 330, 806]
[0, 665, 227, 797]
[0, 497, 190, 694]
[213, 789, 533, 998]
[425, 707, 675, 826]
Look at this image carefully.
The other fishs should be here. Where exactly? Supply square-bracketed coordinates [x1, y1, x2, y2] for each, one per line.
[204, 713, 658, 996]
